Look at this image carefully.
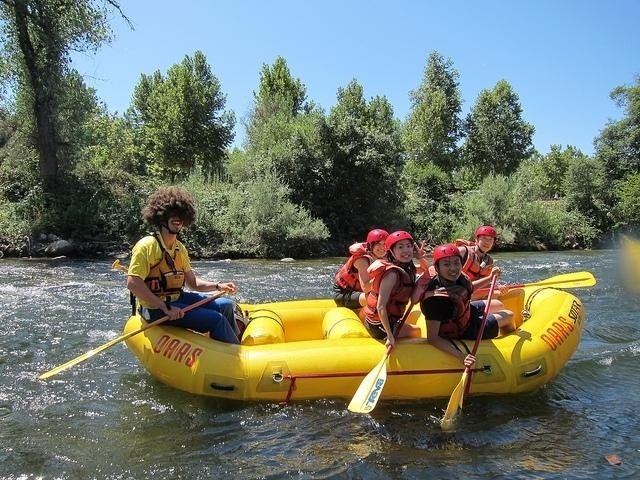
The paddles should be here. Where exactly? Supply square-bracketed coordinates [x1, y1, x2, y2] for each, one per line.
[39, 290, 228, 379]
[346, 265, 437, 413]
[440, 273, 498, 431]
[473, 271, 596, 295]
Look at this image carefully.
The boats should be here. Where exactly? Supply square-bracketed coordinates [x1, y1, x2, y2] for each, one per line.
[124, 285, 583, 403]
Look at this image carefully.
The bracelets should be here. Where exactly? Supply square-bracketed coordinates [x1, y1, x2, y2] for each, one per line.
[416, 250, 426, 260]
[386, 327, 391, 332]
[216, 283, 220, 289]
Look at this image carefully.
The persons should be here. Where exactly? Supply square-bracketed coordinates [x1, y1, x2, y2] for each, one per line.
[459, 225, 510, 301]
[126, 184, 242, 346]
[332, 227, 389, 322]
[363, 231, 430, 352]
[419, 243, 518, 368]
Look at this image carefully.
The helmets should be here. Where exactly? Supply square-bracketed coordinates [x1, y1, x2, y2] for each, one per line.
[473, 226, 497, 241]
[385, 231, 414, 251]
[366, 228, 390, 245]
[433, 243, 462, 265]
